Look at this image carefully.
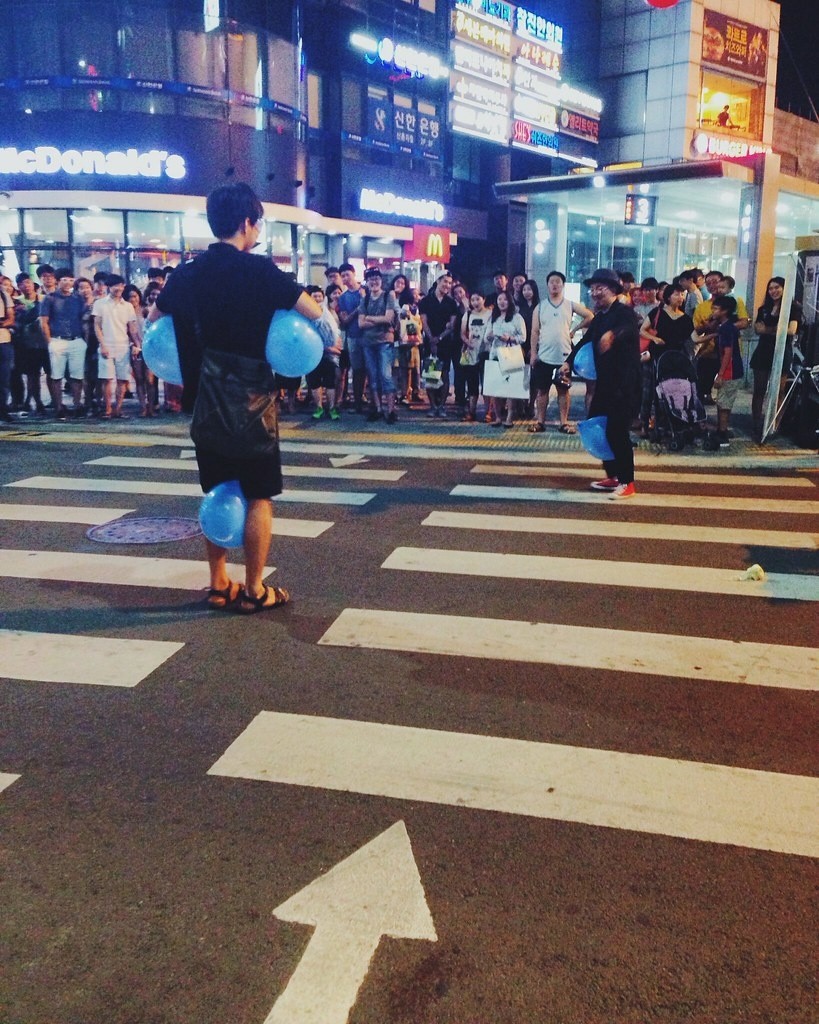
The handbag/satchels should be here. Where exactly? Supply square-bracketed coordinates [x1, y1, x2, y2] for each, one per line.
[482, 359, 531, 400]
[185, 261, 281, 464]
[496, 336, 525, 373]
[637, 307, 660, 353]
[421, 354, 444, 389]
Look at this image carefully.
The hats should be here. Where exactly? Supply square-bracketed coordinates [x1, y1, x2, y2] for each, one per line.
[364, 266, 382, 281]
[432, 269, 452, 284]
[584, 268, 623, 294]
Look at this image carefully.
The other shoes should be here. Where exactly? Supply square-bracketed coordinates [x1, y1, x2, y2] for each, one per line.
[277, 389, 535, 426]
[0, 389, 188, 428]
[627, 414, 742, 455]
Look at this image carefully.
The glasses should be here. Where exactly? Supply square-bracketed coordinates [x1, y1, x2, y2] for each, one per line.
[364, 267, 379, 278]
[587, 286, 609, 293]
[58, 277, 75, 282]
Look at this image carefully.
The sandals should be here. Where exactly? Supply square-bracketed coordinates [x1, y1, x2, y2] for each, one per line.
[204, 578, 289, 612]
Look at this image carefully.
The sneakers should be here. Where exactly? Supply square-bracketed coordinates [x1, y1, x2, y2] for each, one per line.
[528, 422, 546, 433]
[558, 423, 576, 434]
[590, 478, 635, 500]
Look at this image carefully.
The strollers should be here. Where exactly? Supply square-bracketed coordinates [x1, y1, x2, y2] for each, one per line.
[639, 348, 722, 457]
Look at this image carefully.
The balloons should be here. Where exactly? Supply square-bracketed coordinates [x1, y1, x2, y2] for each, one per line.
[197, 480, 249, 548]
[573, 342, 597, 380]
[576, 416, 616, 461]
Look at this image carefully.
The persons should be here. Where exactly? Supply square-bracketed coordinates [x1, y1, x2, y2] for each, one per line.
[623, 271, 636, 303]
[518, 280, 540, 417]
[484, 291, 526, 428]
[511, 271, 528, 304]
[493, 271, 510, 294]
[679, 270, 703, 319]
[693, 271, 748, 397]
[656, 281, 669, 303]
[558, 268, 637, 500]
[710, 297, 744, 446]
[527, 270, 594, 434]
[632, 277, 659, 431]
[418, 270, 460, 417]
[147, 184, 323, 614]
[748, 277, 798, 431]
[613, 270, 627, 305]
[451, 284, 470, 406]
[692, 267, 712, 302]
[1, 262, 429, 425]
[707, 274, 738, 321]
[639, 284, 693, 438]
[627, 287, 641, 310]
[461, 291, 497, 424]
